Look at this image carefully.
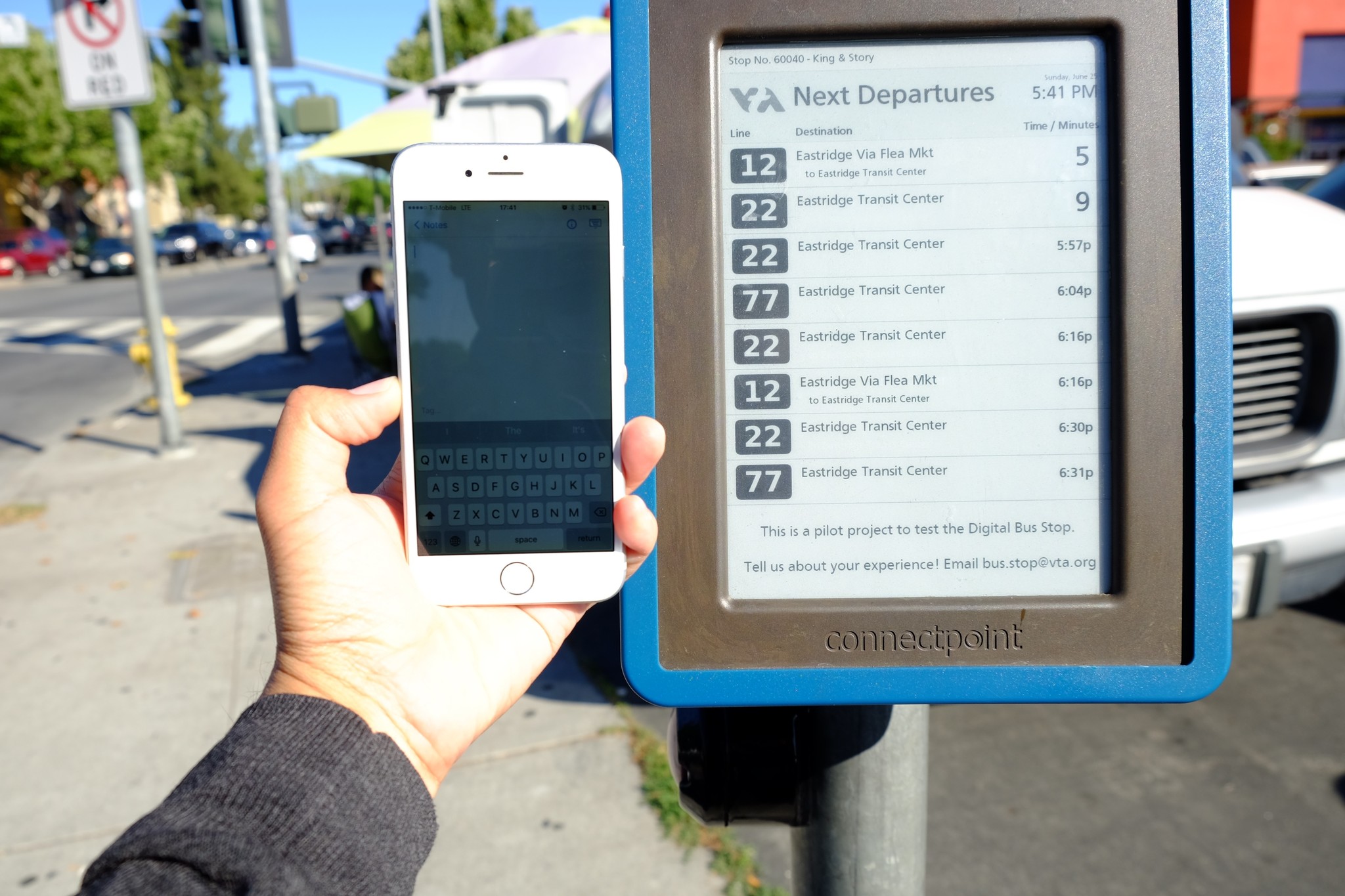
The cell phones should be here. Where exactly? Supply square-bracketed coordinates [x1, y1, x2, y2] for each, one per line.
[390, 142, 630, 604]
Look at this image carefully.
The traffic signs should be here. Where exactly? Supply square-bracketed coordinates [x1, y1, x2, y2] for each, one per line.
[51, 0, 155, 113]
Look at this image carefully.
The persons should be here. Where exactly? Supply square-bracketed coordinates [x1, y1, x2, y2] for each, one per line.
[74, 364, 668, 896]
[349, 266, 398, 376]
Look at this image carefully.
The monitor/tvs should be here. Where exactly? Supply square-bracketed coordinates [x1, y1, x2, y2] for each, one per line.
[715, 37, 1114, 604]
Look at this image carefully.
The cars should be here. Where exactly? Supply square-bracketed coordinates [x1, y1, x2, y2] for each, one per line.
[0, 214, 393, 279]
[1228, 145, 1345, 621]
